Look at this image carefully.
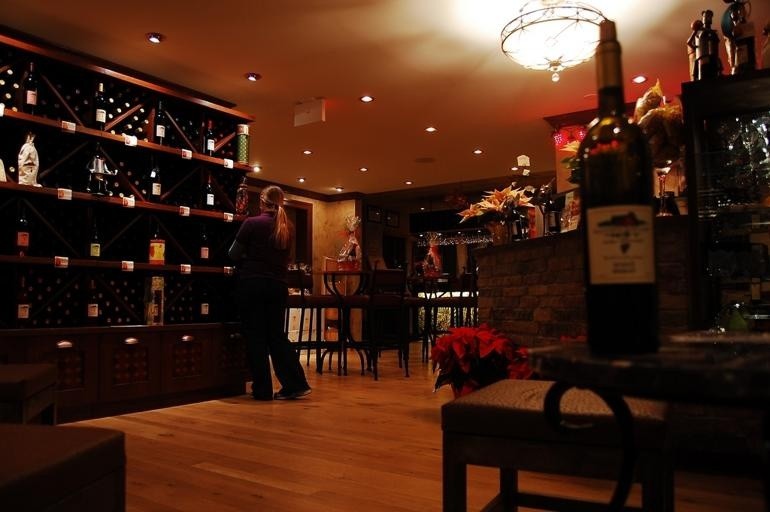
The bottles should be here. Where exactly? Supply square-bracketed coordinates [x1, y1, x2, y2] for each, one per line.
[22, 63, 37, 115]
[204, 175, 215, 210]
[508, 201, 522, 241]
[547, 188, 559, 231]
[149, 221, 165, 266]
[203, 121, 214, 157]
[197, 223, 211, 267]
[155, 101, 166, 144]
[16, 277, 30, 329]
[94, 83, 107, 132]
[88, 216, 101, 259]
[16, 203, 29, 256]
[149, 162, 162, 202]
[199, 282, 210, 322]
[578, 22, 660, 357]
[85, 280, 98, 327]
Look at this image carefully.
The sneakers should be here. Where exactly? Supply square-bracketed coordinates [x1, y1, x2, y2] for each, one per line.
[250, 386, 274, 401]
[274, 384, 312, 401]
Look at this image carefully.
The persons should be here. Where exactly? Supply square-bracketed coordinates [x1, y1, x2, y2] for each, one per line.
[226, 185, 312, 401]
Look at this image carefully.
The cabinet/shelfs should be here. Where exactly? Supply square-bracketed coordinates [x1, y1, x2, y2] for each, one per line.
[0, 34, 254, 425]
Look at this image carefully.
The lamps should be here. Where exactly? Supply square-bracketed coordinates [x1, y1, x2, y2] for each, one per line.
[499, 0, 608, 83]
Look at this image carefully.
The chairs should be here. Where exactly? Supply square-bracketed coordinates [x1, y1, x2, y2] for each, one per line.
[417, 271, 459, 365]
[321, 270, 371, 375]
[284, 268, 315, 366]
[363, 267, 411, 381]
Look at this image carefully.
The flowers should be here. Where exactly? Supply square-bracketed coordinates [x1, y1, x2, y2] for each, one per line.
[426, 325, 508, 392]
[457, 184, 536, 228]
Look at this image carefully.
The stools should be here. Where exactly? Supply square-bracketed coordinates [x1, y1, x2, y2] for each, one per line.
[441, 375, 677, 512]
[0, 425, 124, 512]
[0, 363, 60, 425]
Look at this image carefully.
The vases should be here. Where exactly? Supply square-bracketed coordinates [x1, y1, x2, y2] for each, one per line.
[492, 220, 512, 246]
[450, 380, 480, 398]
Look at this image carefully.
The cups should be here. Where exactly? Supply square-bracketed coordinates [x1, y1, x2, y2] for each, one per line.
[644, 125, 678, 218]
